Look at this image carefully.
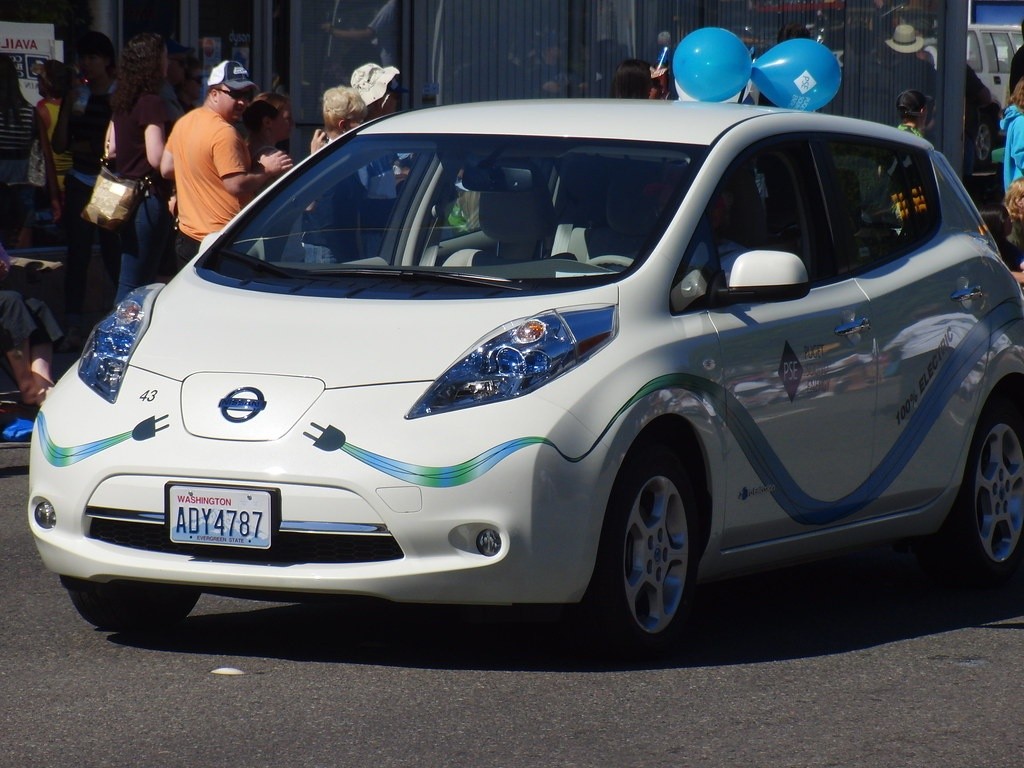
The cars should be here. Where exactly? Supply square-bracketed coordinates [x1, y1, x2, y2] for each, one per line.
[24, 97, 1024, 661]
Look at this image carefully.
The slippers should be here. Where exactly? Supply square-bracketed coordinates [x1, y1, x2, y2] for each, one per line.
[18, 401, 39, 414]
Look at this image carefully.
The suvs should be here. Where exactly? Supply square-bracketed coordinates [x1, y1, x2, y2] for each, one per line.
[969, 25, 1024, 167]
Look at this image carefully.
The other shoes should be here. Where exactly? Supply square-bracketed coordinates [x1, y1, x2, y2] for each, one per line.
[55, 327, 83, 353]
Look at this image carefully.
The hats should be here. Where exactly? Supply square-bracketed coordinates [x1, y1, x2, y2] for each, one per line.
[350, 63, 400, 106]
[896, 91, 933, 117]
[885, 25, 924, 53]
[207, 60, 260, 91]
[166, 41, 195, 55]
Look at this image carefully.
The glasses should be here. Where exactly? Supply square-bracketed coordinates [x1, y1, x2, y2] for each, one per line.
[217, 88, 254, 100]
[168, 58, 184, 66]
[189, 75, 203, 84]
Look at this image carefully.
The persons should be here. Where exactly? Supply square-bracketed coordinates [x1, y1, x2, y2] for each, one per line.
[980, 19, 1024, 286]
[0, 34, 295, 411]
[319, 0, 671, 104]
[760, 0, 991, 196]
[311, 63, 400, 156]
[649, 179, 743, 282]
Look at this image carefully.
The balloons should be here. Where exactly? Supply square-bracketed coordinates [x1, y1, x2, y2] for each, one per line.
[673, 28, 753, 102]
[751, 39, 842, 112]
[675, 79, 751, 102]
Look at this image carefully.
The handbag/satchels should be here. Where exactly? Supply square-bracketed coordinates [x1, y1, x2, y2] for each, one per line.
[28, 107, 48, 190]
[81, 166, 142, 232]
